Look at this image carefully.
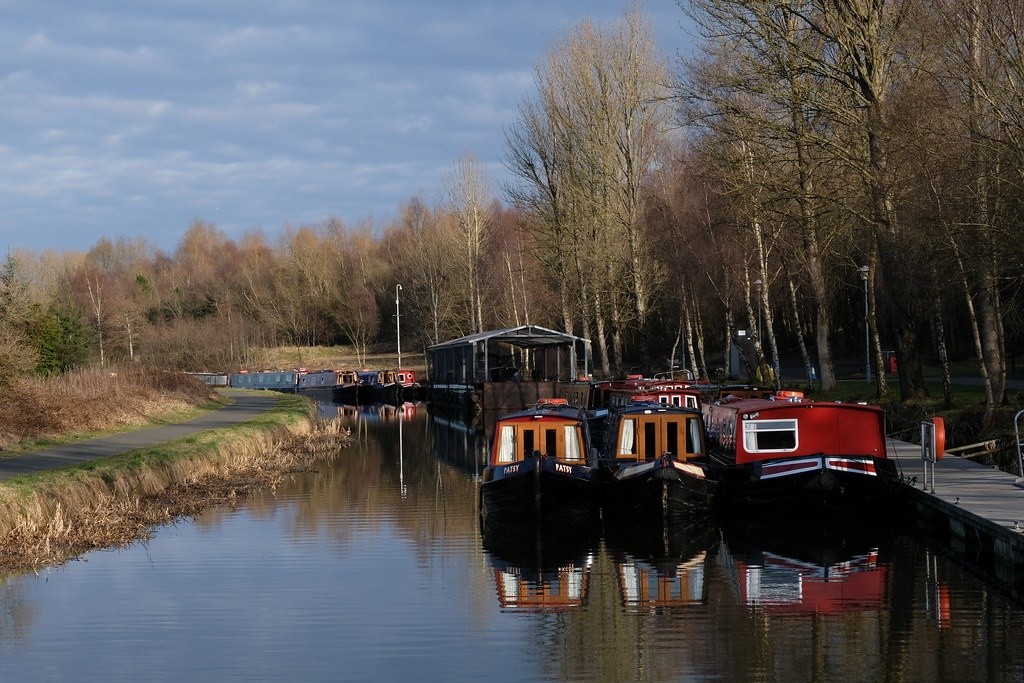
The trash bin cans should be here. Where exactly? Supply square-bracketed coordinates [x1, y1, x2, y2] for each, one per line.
[881, 350, 897, 375]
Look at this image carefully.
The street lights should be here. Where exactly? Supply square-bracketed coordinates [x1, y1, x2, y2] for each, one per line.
[855, 265, 873, 385]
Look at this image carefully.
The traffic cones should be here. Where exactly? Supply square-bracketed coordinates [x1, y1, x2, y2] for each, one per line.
[811, 363, 818, 380]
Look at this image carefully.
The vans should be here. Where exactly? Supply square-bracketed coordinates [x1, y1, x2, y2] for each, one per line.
[882, 350, 898, 373]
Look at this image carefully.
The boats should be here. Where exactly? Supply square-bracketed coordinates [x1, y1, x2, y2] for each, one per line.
[480, 400, 603, 520]
[426, 323, 592, 415]
[332, 369, 421, 408]
[598, 373, 705, 411]
[480, 509, 888, 618]
[700, 391, 900, 524]
[599, 400, 713, 504]
